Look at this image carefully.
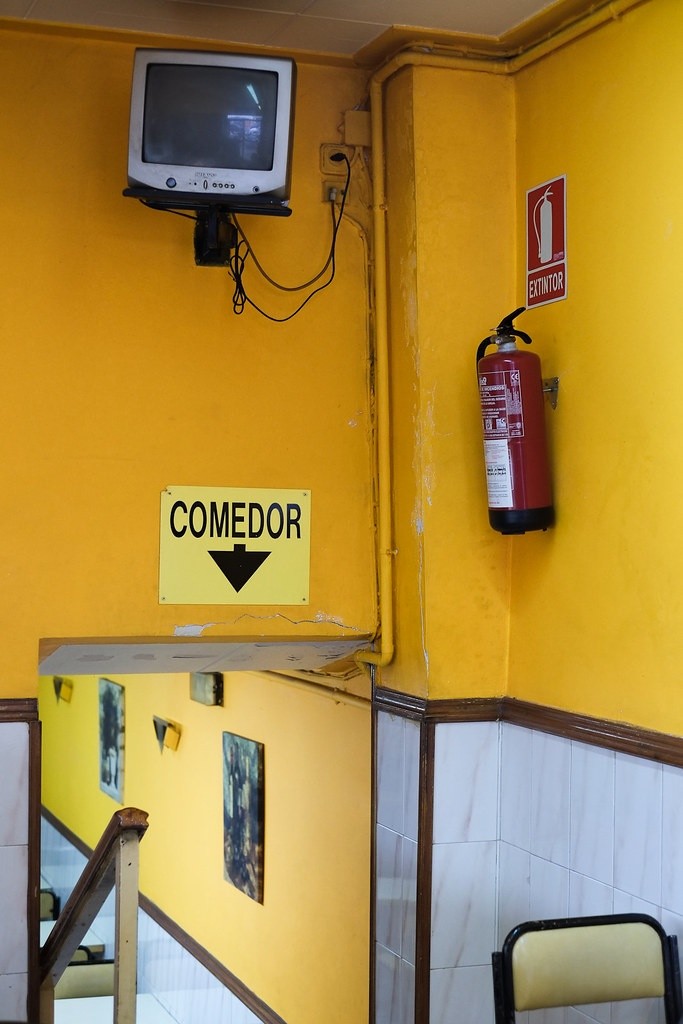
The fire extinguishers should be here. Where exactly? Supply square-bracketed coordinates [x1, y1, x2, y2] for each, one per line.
[472, 304, 558, 537]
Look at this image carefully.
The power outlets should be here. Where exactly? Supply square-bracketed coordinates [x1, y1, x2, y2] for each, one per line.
[323, 180, 350, 203]
[320, 144, 349, 176]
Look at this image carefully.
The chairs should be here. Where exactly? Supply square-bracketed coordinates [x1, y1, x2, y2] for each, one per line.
[490, 913, 683, 1024]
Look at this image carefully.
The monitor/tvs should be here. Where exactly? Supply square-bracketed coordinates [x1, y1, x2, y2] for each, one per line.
[127, 47, 297, 205]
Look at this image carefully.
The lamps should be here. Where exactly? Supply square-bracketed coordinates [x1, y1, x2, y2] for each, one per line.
[190, 672, 224, 706]
